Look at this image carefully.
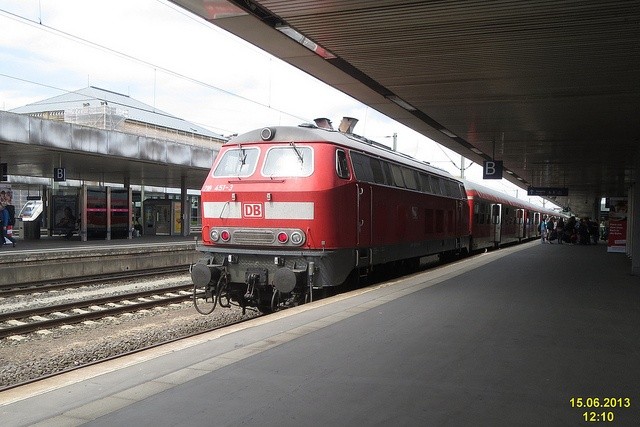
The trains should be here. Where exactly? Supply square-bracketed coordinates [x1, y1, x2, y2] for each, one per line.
[190, 117, 572, 315]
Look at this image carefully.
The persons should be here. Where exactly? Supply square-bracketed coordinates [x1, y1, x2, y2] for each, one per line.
[537, 214, 602, 244]
[5, 190, 13, 205]
[56, 206, 77, 227]
[0, 202, 17, 247]
[0, 190, 7, 205]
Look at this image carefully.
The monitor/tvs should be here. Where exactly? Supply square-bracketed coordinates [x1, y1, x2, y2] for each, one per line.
[20, 202, 40, 218]
